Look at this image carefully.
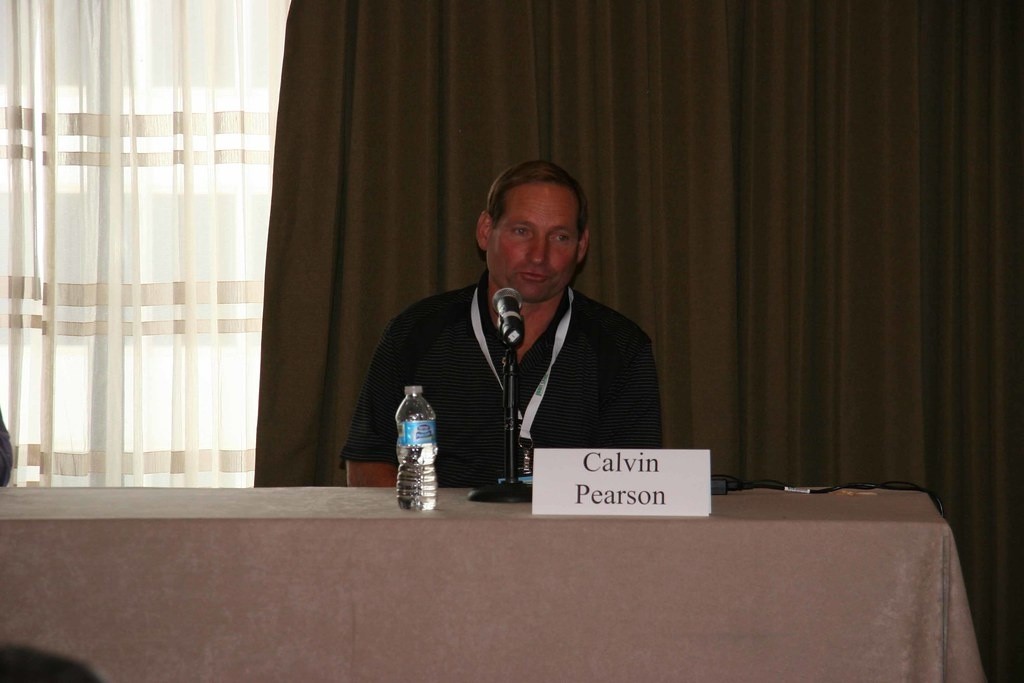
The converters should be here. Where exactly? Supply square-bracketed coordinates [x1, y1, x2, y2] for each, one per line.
[711, 478, 728, 495]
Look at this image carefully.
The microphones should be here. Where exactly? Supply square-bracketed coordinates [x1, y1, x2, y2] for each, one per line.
[492, 287, 526, 346]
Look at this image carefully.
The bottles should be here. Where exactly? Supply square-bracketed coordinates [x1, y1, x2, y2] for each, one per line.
[395, 386, 439, 511]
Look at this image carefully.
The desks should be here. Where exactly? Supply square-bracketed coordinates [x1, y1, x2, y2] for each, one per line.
[0, 487, 991, 683]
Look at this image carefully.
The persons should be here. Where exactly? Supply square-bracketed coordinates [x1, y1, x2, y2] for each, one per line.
[339, 159, 661, 488]
